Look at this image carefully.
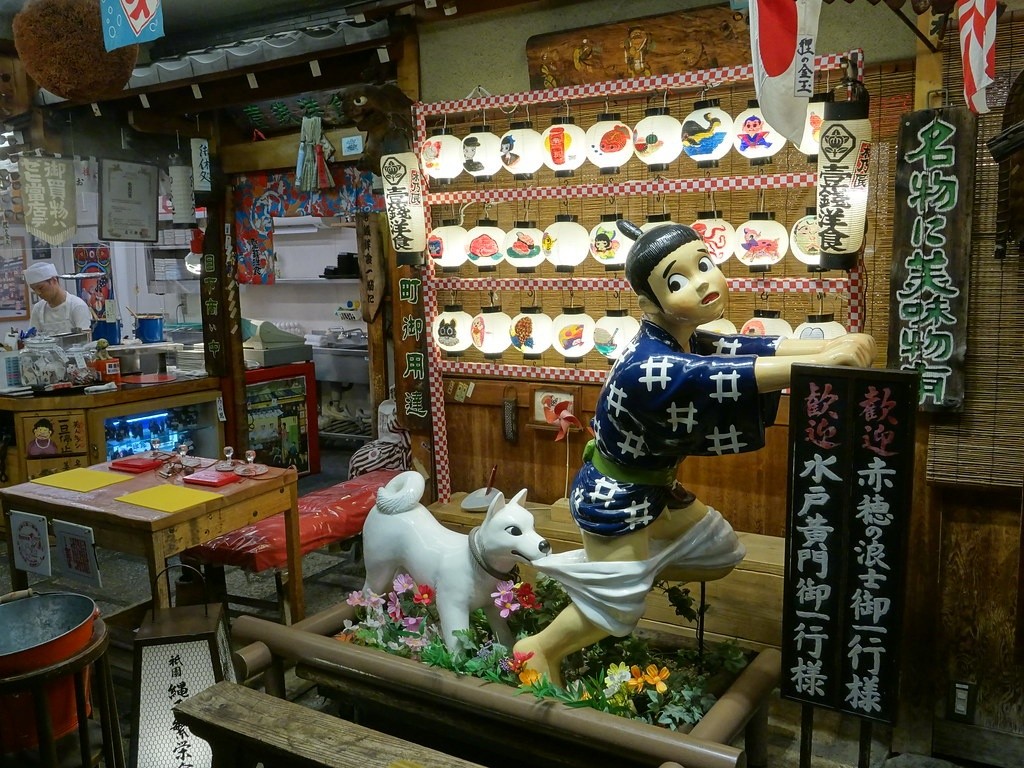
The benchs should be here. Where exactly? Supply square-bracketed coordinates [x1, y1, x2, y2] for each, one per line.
[427, 491, 785, 647]
[174, 680, 486, 768]
[179, 469, 402, 626]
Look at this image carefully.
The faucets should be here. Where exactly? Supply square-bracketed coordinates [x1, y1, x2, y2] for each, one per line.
[325, 326, 365, 340]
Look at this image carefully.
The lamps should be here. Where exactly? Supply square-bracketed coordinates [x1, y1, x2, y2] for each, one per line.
[816, 79, 872, 269]
[380, 130, 427, 266]
[188, 114, 213, 196]
[128, 564, 241, 768]
[168, 131, 198, 230]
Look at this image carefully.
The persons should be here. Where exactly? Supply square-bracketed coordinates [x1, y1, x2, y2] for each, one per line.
[24, 262, 92, 337]
[512, 220, 882, 689]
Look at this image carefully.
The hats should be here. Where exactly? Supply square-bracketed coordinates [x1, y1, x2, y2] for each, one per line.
[24, 262, 58, 285]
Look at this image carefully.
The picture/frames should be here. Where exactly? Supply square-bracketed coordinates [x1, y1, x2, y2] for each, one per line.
[0, 235, 30, 322]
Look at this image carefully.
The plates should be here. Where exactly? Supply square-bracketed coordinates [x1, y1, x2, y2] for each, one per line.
[461, 487, 501, 512]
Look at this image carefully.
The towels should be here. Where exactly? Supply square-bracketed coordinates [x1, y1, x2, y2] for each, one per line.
[302, 333, 327, 347]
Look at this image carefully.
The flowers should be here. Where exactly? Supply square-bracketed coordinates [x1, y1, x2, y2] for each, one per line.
[339, 572, 749, 734]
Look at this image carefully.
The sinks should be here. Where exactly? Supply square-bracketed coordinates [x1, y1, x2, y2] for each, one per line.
[311, 343, 369, 384]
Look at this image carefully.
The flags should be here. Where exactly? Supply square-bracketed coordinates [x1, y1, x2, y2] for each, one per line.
[957, 0, 998, 116]
[746, 0, 821, 148]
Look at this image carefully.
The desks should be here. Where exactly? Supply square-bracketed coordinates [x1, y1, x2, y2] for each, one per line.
[0, 450, 305, 623]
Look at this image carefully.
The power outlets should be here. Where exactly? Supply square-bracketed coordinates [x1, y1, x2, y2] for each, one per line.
[180, 298, 186, 309]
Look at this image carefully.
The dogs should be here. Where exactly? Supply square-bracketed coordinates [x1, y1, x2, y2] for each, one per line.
[360, 469, 553, 665]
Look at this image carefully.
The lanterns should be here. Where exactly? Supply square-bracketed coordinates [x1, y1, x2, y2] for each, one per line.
[815, 100, 871, 271]
[127, 603, 241, 768]
[422, 96, 847, 366]
[379, 135, 425, 264]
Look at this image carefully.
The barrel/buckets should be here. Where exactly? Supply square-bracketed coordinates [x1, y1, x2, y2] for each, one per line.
[90, 318, 123, 345]
[0, 592, 99, 750]
[135, 316, 163, 343]
